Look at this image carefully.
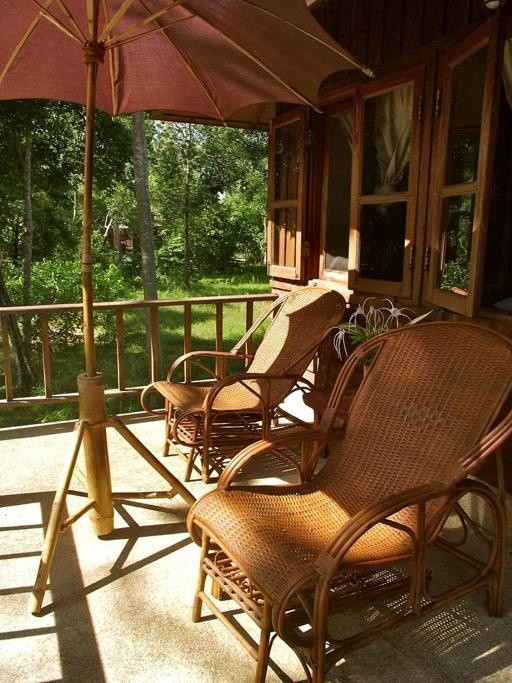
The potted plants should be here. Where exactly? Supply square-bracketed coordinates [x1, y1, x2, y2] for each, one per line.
[324, 296, 432, 380]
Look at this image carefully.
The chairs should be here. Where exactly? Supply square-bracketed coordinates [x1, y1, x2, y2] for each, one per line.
[140, 286, 345, 482]
[185, 321, 511, 683]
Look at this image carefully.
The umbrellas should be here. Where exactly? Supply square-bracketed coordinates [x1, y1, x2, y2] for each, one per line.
[0, 0, 376, 377]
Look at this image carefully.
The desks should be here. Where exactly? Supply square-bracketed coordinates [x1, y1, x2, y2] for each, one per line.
[303, 385, 360, 458]
